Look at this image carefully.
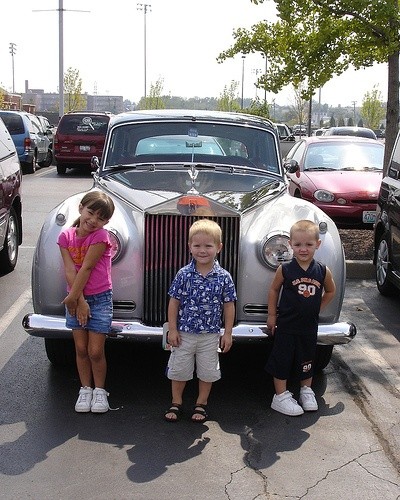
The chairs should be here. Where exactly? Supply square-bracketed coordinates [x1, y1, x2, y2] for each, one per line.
[307, 155, 324, 168]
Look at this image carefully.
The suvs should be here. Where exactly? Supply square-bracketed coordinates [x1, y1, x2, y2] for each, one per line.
[0, 111, 53, 173]
[51, 112, 116, 175]
[372, 128, 400, 297]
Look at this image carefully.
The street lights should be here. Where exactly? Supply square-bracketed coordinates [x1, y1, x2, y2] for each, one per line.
[136, 2, 152, 100]
[8, 42, 18, 94]
[241, 55, 248, 110]
[30, 0, 92, 117]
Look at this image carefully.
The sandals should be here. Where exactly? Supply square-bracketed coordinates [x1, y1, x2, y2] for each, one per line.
[191, 404, 209, 422]
[164, 402, 182, 421]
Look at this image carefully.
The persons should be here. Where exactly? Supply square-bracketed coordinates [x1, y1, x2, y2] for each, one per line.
[161, 218, 238, 423]
[56, 191, 115, 413]
[267, 220, 336, 417]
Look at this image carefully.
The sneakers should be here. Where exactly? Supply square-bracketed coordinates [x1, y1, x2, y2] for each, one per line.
[270, 390, 304, 416]
[299, 386, 318, 410]
[74, 386, 92, 412]
[90, 388, 119, 412]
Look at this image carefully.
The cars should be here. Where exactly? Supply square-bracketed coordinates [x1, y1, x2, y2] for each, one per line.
[281, 136, 385, 229]
[274, 123, 296, 160]
[0, 117, 24, 272]
[313, 127, 384, 134]
[292, 124, 307, 137]
[20, 108, 356, 376]
[37, 115, 57, 143]
